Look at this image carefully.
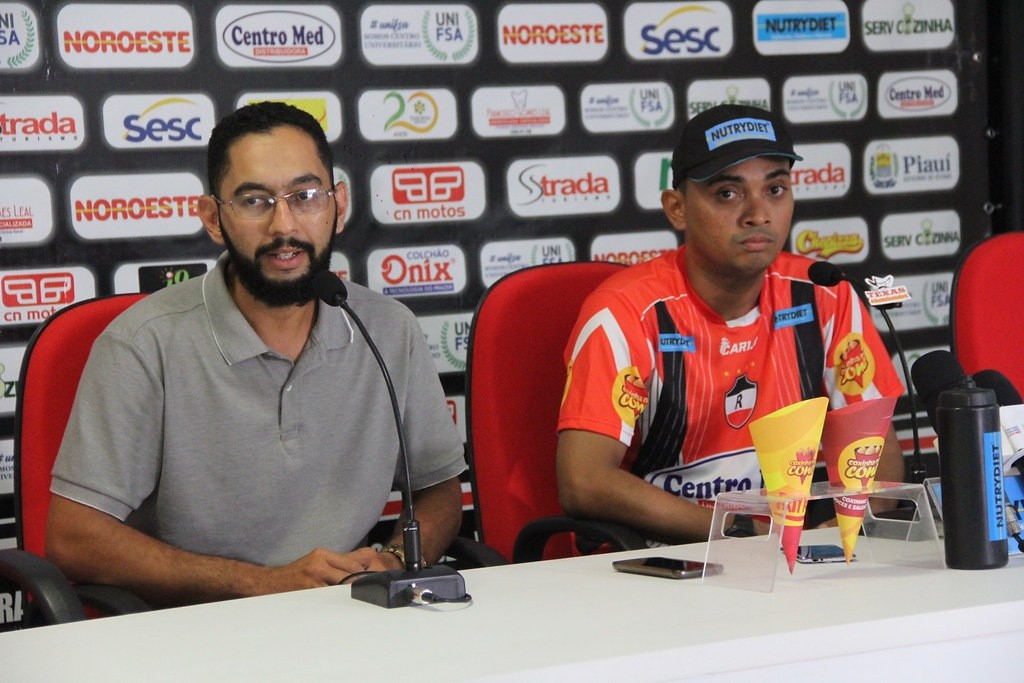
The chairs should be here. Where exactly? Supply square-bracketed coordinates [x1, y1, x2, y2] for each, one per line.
[464, 259, 651, 564]
[11, 292, 507, 630]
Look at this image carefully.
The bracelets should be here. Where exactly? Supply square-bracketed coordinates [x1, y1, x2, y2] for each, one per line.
[378, 543, 427, 569]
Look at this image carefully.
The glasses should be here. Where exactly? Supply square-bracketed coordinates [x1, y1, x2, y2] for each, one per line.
[211, 186, 338, 221]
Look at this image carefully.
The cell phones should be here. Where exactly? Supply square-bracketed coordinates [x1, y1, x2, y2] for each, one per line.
[780, 544, 858, 564]
[612, 556, 724, 581]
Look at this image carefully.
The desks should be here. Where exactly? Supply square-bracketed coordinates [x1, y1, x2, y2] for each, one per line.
[0, 508, 1024, 683]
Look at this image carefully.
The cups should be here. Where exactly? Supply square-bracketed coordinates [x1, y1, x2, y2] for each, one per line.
[936, 388, 1008, 570]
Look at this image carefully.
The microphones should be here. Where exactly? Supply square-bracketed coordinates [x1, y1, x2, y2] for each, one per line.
[970, 369, 1024, 486]
[911, 349, 1019, 541]
[313, 267, 464, 609]
[808, 262, 943, 538]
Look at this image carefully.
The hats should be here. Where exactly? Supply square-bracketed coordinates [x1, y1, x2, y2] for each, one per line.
[672, 104, 804, 191]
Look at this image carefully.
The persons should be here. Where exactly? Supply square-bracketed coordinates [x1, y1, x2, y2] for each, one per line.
[45, 101, 470, 596]
[555, 103, 905, 541]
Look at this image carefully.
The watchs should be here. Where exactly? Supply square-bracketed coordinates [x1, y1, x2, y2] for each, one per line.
[725, 513, 758, 537]
